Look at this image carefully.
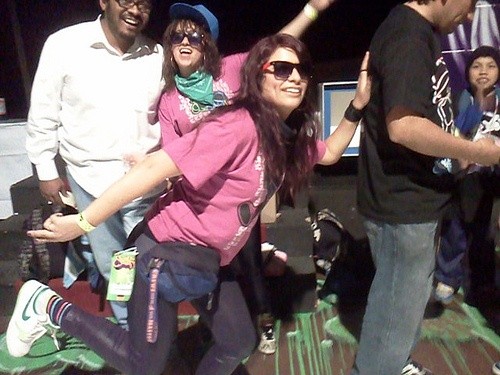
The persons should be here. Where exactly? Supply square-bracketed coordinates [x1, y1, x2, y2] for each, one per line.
[23, 0, 169, 334]
[355, 0, 500, 375]
[119, 0, 338, 356]
[5, 34, 374, 375]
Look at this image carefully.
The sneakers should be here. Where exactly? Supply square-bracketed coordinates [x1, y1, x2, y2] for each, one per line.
[259, 325, 278, 354]
[6, 279, 61, 358]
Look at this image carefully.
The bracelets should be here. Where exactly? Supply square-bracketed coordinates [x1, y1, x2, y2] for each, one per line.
[74, 210, 97, 232]
[344, 99, 368, 121]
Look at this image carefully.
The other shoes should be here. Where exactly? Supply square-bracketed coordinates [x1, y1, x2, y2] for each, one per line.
[492, 359, 500, 375]
[435, 282, 454, 298]
[401, 360, 434, 375]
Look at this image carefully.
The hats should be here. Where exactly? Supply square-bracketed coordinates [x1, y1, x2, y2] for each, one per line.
[169, 3, 219, 43]
[466, 45, 500, 85]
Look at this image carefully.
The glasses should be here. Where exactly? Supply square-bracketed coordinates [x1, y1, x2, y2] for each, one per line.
[171, 30, 205, 43]
[115, 0, 152, 13]
[262, 61, 313, 80]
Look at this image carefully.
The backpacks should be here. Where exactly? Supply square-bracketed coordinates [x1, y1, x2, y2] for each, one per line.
[310, 207, 369, 274]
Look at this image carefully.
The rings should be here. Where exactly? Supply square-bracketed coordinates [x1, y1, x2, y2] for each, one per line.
[359, 69, 369, 72]
[47, 201, 52, 205]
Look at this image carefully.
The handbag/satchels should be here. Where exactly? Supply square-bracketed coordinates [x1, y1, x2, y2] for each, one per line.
[139, 241, 221, 343]
[19, 203, 79, 285]
